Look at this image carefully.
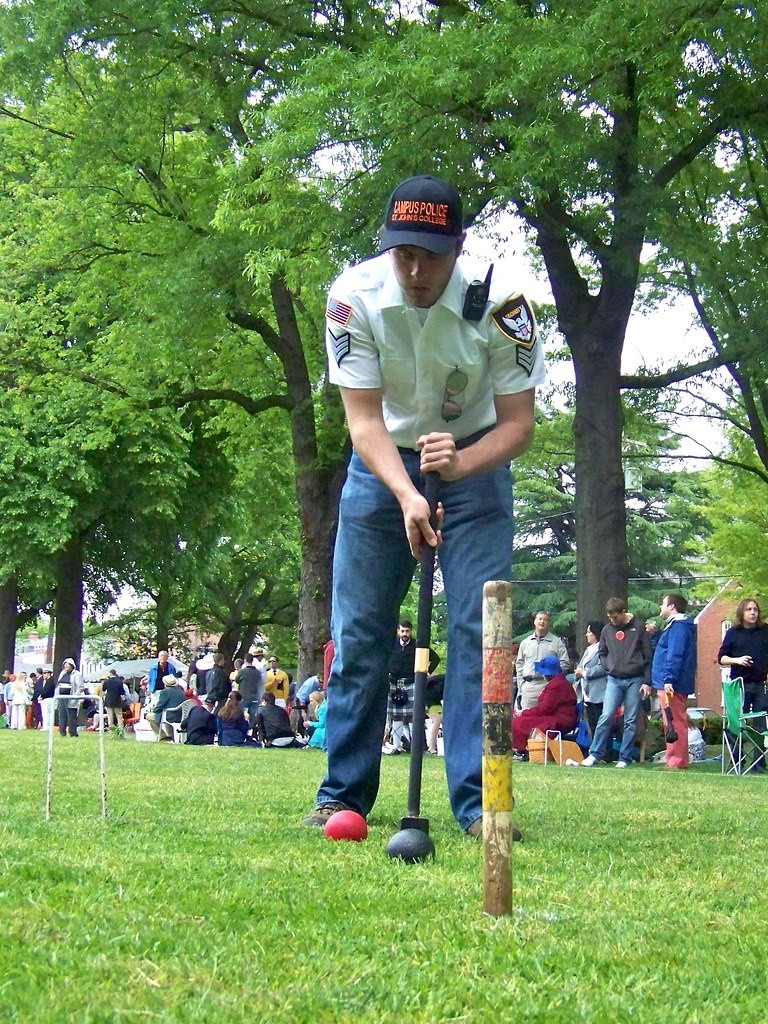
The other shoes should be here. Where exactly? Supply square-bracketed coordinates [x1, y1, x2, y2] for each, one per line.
[728, 763, 743, 773]
[750, 761, 768, 774]
[582, 754, 599, 767]
[60, 730, 65, 736]
[615, 761, 626, 768]
[512, 749, 528, 761]
[69, 731, 78, 737]
[86, 725, 98, 730]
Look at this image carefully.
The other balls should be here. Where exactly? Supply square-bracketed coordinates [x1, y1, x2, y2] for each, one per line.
[385, 828, 435, 863]
[323, 810, 367, 842]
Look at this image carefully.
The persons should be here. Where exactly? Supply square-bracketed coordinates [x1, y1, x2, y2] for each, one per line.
[320, 627, 335, 692]
[719, 599, 768, 773]
[515, 593, 698, 771]
[53, 657, 84, 737]
[144, 645, 305, 748]
[0, 667, 55, 730]
[305, 171, 546, 837]
[296, 672, 327, 751]
[81, 688, 99, 718]
[381, 620, 446, 754]
[92, 670, 139, 734]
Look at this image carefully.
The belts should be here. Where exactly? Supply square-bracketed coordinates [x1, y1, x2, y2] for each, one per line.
[524, 676, 545, 682]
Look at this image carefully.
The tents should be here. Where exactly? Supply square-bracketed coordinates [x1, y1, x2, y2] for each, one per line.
[84, 656, 190, 683]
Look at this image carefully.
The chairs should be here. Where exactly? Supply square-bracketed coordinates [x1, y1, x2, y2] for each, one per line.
[158, 698, 198, 744]
[123, 703, 141, 732]
[721, 677, 768, 776]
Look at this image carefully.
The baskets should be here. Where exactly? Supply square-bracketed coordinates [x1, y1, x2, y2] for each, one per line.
[525, 738, 554, 765]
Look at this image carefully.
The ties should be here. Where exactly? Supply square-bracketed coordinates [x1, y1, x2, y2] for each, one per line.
[272, 670, 276, 675]
[402, 643, 407, 650]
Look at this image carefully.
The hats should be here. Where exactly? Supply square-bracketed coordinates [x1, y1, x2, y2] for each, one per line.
[535, 656, 563, 675]
[43, 668, 53, 673]
[162, 674, 178, 687]
[205, 694, 216, 705]
[1, 670, 11, 678]
[379, 176, 462, 254]
[251, 649, 263, 656]
[196, 654, 215, 671]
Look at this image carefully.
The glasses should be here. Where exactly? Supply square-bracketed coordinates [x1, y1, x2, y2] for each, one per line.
[441, 368, 468, 424]
[254, 655, 261, 657]
[269, 660, 277, 663]
[319, 680, 323, 683]
[44, 672, 48, 674]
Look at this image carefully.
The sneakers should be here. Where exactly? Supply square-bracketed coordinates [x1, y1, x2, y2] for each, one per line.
[468, 817, 521, 845]
[306, 801, 351, 826]
